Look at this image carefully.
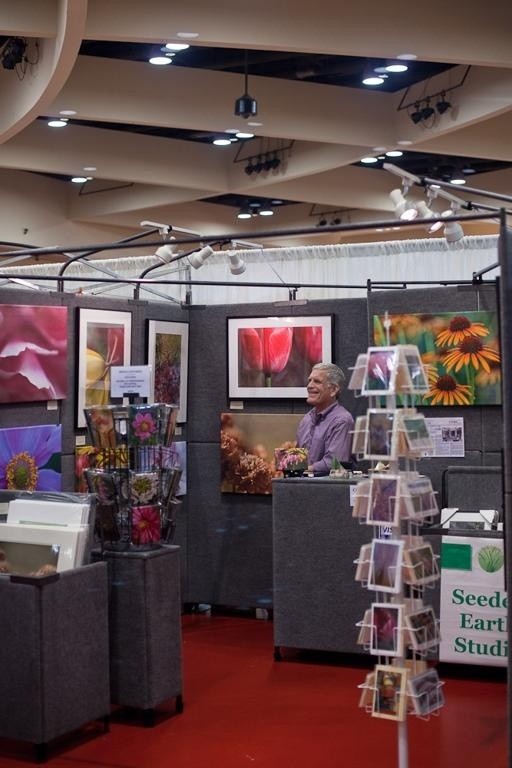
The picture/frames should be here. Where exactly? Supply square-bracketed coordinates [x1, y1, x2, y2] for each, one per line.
[442, 513, 493, 530]
[0, 500, 91, 577]
[348, 343, 444, 720]
[73, 306, 135, 432]
[144, 319, 192, 426]
[226, 313, 334, 402]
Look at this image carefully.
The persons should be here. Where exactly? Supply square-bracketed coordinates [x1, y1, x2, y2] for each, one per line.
[294, 362, 356, 477]
[379, 677, 396, 710]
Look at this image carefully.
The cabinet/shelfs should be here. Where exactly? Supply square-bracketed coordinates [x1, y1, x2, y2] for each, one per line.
[93, 544, 182, 727]
[0, 562, 112, 763]
[271, 476, 378, 660]
